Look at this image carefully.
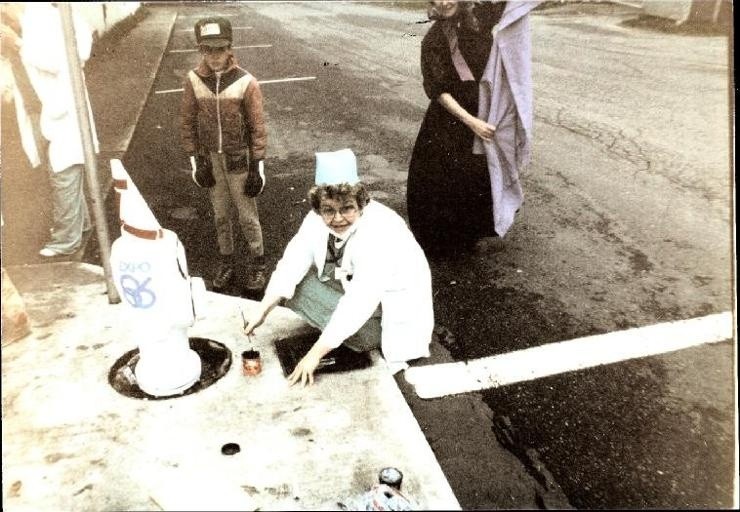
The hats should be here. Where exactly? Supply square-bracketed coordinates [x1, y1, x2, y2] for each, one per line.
[195, 16, 233, 48]
[313, 147, 357, 185]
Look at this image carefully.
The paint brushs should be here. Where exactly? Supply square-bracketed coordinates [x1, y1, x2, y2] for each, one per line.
[240, 310, 253, 351]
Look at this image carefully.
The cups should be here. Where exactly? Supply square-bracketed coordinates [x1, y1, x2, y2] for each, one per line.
[241, 350, 262, 376]
[379, 467, 403, 489]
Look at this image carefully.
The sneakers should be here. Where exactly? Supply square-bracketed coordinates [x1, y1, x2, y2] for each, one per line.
[247, 266, 265, 292]
[38, 247, 60, 256]
[213, 263, 235, 288]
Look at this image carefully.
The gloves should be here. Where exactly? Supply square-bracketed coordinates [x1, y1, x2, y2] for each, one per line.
[189, 156, 216, 188]
[244, 159, 266, 198]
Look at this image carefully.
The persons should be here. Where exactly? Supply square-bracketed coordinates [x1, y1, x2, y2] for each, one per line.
[240, 149, 434, 389]
[0, 2, 100, 260]
[182, 16, 269, 291]
[407, 1, 508, 254]
